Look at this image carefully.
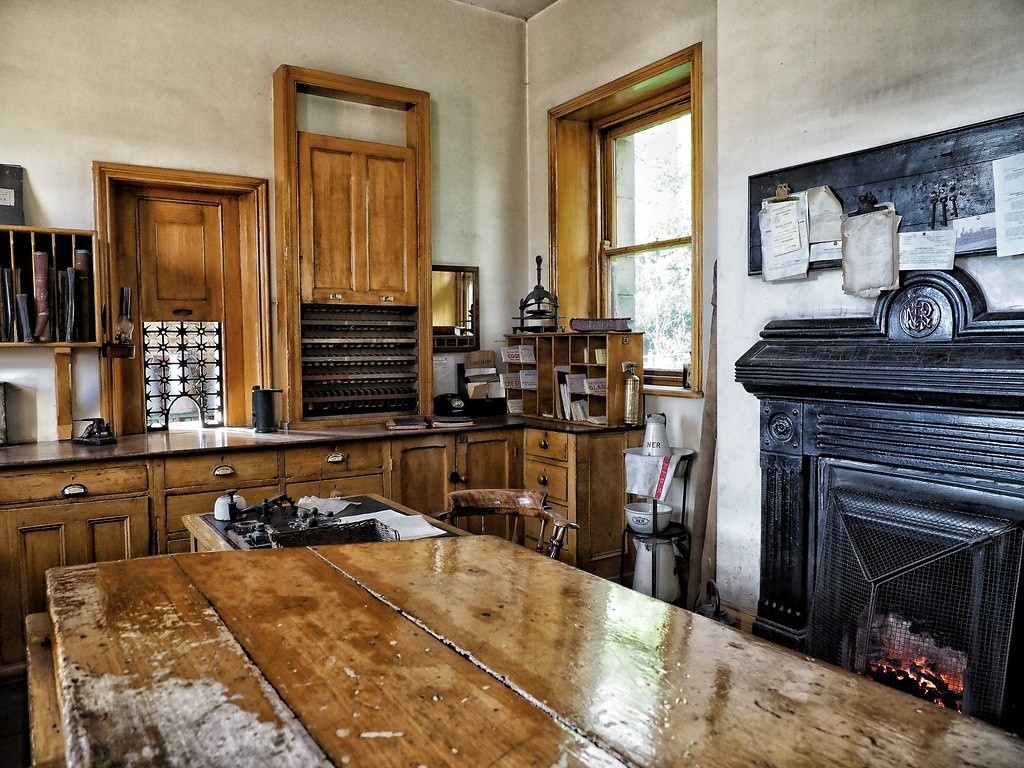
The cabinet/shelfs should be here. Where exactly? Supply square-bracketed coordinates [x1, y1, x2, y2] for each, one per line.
[504, 331, 645, 429]
[620, 449, 695, 610]
[0, 459, 152, 677]
[298, 131, 420, 308]
[154, 439, 391, 558]
[390, 426, 523, 548]
[0, 225, 102, 348]
[523, 426, 647, 589]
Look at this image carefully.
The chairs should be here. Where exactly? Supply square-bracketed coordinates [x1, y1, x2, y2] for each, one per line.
[429, 489, 580, 561]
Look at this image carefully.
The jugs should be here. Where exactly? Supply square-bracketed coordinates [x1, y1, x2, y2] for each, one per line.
[631, 537, 688, 603]
[694, 579, 737, 627]
[642, 413, 671, 457]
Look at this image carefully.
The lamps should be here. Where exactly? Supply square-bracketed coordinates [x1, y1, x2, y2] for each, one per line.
[99, 285, 136, 359]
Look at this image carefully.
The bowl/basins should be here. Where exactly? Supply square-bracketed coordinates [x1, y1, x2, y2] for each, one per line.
[213, 495, 247, 521]
[624, 503, 674, 533]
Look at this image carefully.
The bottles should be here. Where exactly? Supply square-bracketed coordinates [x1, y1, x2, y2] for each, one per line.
[624, 372, 640, 424]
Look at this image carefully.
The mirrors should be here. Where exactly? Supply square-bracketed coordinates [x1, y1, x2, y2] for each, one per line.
[432, 265, 480, 353]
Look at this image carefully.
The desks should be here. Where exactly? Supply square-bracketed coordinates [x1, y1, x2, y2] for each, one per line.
[180, 491, 476, 553]
[46, 534, 1024, 768]
